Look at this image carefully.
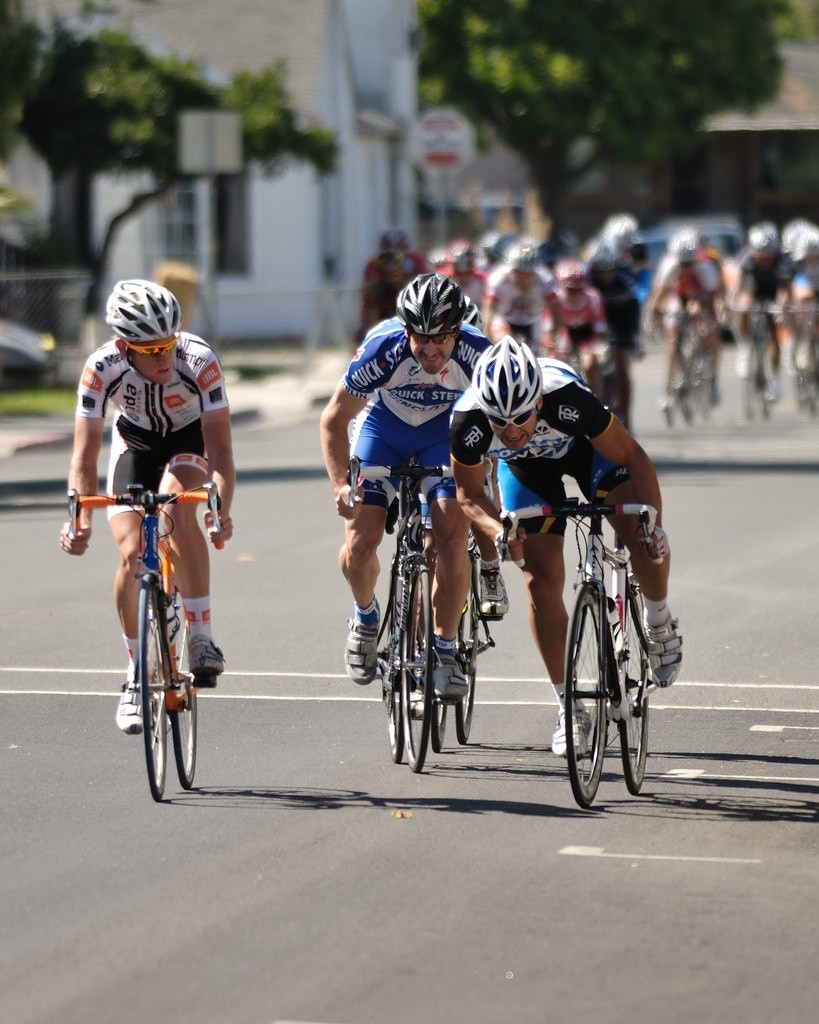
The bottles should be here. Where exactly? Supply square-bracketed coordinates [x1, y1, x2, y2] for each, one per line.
[608, 597, 623, 652]
[163, 593, 179, 647]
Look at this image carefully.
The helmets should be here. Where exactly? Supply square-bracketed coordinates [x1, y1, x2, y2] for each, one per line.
[380, 213, 819, 291]
[472, 336, 543, 418]
[104, 277, 182, 342]
[396, 272, 465, 336]
[463, 295, 483, 333]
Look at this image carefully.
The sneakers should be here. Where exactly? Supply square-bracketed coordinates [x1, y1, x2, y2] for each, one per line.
[344, 599, 382, 686]
[479, 568, 509, 614]
[188, 633, 225, 688]
[644, 611, 683, 688]
[552, 707, 592, 757]
[432, 654, 468, 699]
[116, 682, 143, 734]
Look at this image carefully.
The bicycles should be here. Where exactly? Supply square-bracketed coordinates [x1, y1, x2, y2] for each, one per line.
[61, 480, 222, 804]
[516, 290, 819, 441]
[341, 454, 498, 773]
[494, 498, 671, 809]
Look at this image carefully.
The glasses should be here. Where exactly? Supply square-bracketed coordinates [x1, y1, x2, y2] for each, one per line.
[488, 410, 533, 429]
[122, 330, 182, 358]
[409, 329, 458, 345]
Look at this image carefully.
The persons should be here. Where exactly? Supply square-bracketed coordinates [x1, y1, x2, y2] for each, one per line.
[58, 277, 235, 735]
[318, 270, 686, 758]
[357, 213, 819, 405]
